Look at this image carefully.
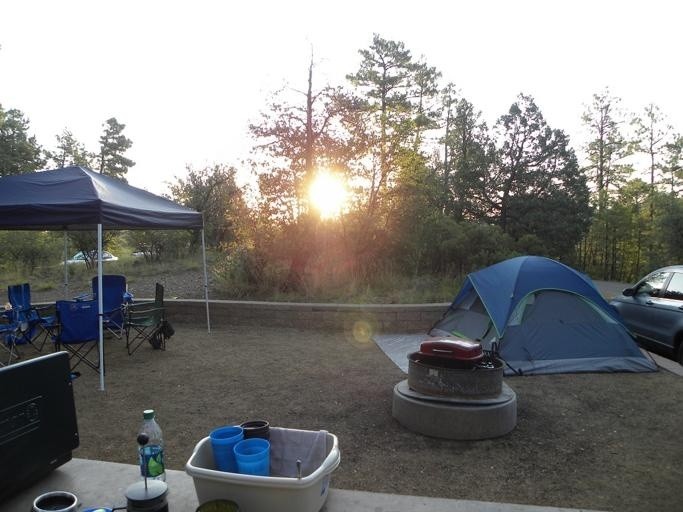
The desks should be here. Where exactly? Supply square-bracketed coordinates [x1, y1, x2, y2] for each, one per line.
[0, 457, 600, 512]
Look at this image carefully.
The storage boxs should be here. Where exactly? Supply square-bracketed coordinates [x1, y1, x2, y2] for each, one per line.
[417, 336, 485, 369]
[185, 425, 341, 512]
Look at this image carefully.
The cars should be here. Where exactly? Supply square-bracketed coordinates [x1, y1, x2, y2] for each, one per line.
[61, 249, 119, 265]
[607, 265, 682, 365]
[130, 242, 161, 257]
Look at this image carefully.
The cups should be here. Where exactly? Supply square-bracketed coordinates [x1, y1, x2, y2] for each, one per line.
[240, 420, 273, 442]
[234, 438, 271, 477]
[211, 424, 244, 470]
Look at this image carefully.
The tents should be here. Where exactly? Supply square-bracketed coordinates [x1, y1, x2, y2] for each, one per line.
[426, 256, 659, 376]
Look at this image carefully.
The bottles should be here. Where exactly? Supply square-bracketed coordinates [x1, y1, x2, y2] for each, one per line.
[136, 410, 166, 482]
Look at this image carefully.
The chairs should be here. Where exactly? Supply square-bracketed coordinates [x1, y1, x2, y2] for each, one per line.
[0, 274, 169, 376]
